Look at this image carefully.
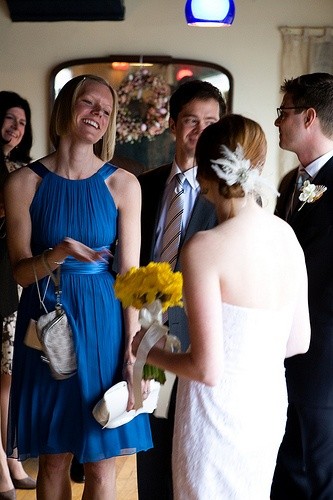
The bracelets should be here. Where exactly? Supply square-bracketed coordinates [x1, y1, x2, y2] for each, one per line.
[44, 248, 65, 264]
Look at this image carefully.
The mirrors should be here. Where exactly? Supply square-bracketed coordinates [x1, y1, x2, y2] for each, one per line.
[48, 51, 233, 177]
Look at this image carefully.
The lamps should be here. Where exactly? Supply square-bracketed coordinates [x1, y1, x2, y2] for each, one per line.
[185, 0, 236, 27]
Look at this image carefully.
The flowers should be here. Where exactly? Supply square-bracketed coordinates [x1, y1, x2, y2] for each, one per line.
[115, 70, 172, 144]
[114, 262, 185, 385]
[298, 176, 326, 210]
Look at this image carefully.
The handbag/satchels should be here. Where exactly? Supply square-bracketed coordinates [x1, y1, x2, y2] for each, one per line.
[37, 309, 77, 379]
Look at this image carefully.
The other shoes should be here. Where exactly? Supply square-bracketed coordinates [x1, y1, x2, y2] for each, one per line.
[12, 478, 37, 489]
[0, 489, 16, 499]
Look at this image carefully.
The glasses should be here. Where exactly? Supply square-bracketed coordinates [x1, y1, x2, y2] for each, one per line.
[277, 107, 318, 117]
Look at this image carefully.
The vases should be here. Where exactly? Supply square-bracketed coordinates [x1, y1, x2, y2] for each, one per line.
[126, 99, 152, 122]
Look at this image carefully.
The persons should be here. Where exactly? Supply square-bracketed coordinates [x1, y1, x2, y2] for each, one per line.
[0, 90, 37, 500]
[7, 75, 154, 500]
[136, 80, 228, 500]
[269, 72, 333, 500]
[131, 113, 312, 500]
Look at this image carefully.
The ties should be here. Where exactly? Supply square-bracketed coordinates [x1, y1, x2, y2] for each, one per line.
[287, 169, 309, 223]
[157, 173, 186, 297]
